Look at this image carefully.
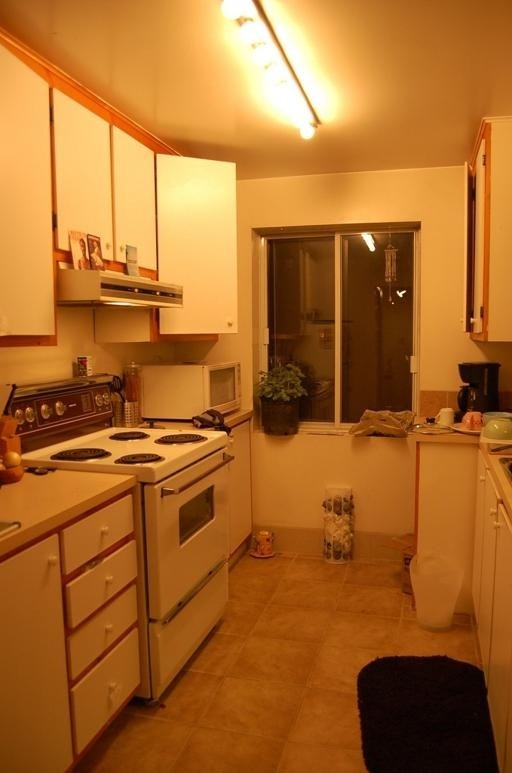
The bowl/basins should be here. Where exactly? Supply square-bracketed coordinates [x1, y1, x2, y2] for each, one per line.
[483, 411, 512, 441]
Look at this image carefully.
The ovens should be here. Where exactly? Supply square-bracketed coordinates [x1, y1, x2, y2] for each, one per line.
[144, 446, 230, 698]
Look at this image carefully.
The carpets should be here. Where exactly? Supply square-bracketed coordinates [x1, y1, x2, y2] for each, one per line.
[356, 653, 499, 772]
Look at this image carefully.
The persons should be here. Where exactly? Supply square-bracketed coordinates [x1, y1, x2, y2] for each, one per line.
[91, 239, 104, 268]
[76, 237, 89, 269]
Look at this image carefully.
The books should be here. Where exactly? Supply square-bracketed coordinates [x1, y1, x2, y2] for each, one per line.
[124, 244, 141, 277]
[68, 230, 90, 269]
[87, 234, 106, 271]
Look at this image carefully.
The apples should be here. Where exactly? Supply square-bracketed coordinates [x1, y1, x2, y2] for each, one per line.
[0, 451, 21, 470]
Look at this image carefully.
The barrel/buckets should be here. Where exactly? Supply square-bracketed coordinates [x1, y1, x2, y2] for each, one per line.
[111, 401, 139, 428]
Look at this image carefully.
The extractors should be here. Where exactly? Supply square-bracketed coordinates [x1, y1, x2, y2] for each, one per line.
[56, 261, 184, 309]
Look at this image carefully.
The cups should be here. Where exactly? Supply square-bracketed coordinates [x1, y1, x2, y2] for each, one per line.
[463, 412, 482, 429]
[434, 407, 455, 428]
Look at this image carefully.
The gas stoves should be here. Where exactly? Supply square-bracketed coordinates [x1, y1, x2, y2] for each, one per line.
[18, 425, 228, 483]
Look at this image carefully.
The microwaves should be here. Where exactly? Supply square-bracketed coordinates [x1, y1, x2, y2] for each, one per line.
[139, 360, 243, 419]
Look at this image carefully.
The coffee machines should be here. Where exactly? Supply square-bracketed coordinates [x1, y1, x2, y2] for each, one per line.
[456, 362, 502, 424]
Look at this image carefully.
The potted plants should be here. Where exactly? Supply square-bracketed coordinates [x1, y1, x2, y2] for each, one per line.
[256, 359, 306, 436]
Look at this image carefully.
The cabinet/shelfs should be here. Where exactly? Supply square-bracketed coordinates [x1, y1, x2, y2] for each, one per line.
[461, 114, 512, 342]
[0, 42, 57, 346]
[93, 147, 238, 344]
[0, 491, 142, 772]
[471, 449, 486, 627]
[477, 467, 511, 773]
[47, 87, 157, 273]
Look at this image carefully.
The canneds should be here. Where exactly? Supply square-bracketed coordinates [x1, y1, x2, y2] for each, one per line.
[77, 356, 94, 377]
